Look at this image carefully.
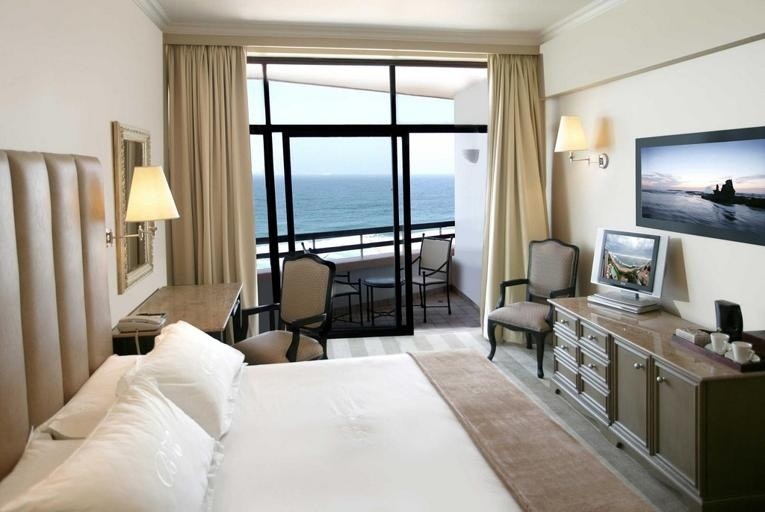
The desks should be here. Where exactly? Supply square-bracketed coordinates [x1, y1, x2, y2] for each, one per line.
[112, 282, 245, 355]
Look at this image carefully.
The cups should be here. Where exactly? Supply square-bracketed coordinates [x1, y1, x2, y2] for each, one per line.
[732, 341, 754, 362]
[711, 333, 731, 352]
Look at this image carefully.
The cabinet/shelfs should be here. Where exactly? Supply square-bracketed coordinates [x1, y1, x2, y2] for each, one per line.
[546, 297, 765, 511]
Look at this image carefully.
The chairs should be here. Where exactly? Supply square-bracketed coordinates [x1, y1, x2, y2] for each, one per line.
[400, 233, 453, 324]
[230, 253, 337, 366]
[301, 242, 364, 328]
[487, 238, 579, 379]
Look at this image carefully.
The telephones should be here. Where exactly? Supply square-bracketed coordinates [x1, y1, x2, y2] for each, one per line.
[118, 315, 166, 333]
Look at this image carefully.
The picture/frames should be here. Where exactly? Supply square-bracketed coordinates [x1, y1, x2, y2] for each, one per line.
[636, 126, 765, 247]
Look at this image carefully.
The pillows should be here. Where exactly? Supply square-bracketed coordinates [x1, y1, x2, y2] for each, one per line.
[0, 438, 89, 512]
[0, 374, 226, 512]
[49, 355, 147, 439]
[115, 319, 249, 441]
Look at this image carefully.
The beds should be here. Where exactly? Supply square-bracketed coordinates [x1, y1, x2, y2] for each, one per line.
[0, 150, 654, 512]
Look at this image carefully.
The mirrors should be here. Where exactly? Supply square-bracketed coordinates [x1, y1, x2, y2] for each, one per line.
[112, 121, 154, 296]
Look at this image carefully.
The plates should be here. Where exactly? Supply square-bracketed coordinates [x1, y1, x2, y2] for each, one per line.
[724, 351, 760, 364]
[705, 343, 732, 355]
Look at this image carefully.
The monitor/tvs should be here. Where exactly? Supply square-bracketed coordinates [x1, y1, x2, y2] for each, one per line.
[588, 227, 669, 315]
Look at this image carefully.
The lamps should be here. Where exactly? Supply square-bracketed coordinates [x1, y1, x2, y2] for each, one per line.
[105, 166, 180, 247]
[554, 116, 608, 169]
